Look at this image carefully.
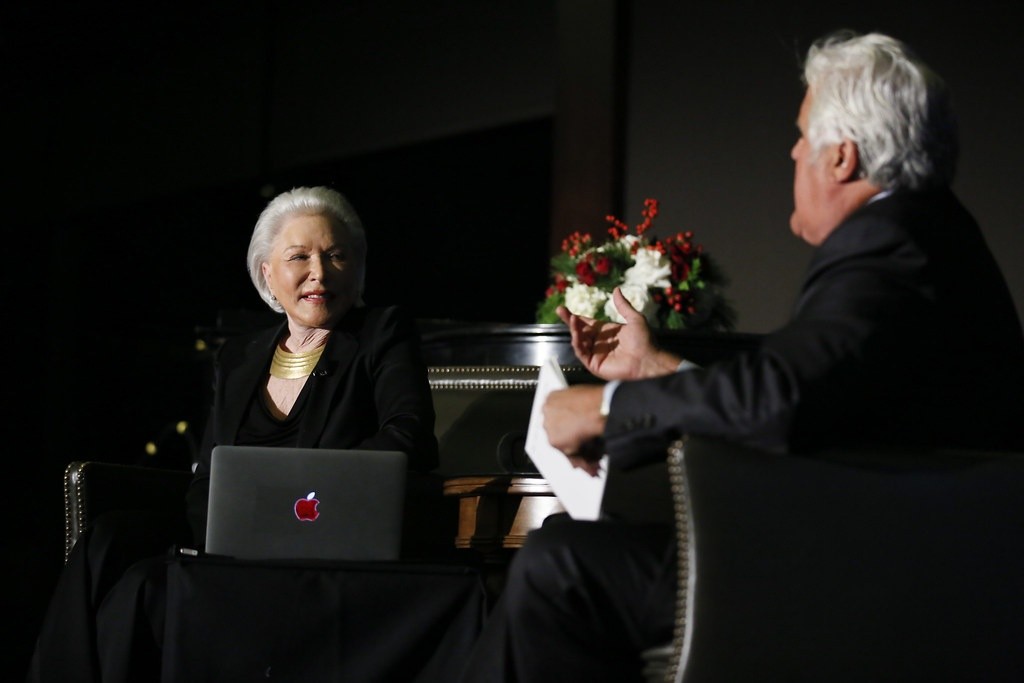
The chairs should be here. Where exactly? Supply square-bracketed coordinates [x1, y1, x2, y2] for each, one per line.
[61, 459, 203, 571]
[625, 433, 1023, 683]
[416, 364, 539, 548]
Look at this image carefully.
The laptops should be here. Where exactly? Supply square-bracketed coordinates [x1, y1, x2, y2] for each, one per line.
[181, 445, 407, 562]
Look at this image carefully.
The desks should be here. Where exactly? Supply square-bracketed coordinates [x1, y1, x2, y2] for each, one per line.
[91, 541, 516, 683]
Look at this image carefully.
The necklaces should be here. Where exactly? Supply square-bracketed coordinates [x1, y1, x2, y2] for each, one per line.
[270, 341, 325, 381]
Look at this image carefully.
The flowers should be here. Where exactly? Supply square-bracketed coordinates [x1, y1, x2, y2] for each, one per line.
[535, 195, 738, 337]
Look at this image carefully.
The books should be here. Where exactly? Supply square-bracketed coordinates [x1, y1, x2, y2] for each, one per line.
[524, 356, 609, 521]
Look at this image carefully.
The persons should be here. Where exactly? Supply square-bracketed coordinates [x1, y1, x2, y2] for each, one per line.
[29, 188, 439, 683]
[502, 30, 1024, 683]
